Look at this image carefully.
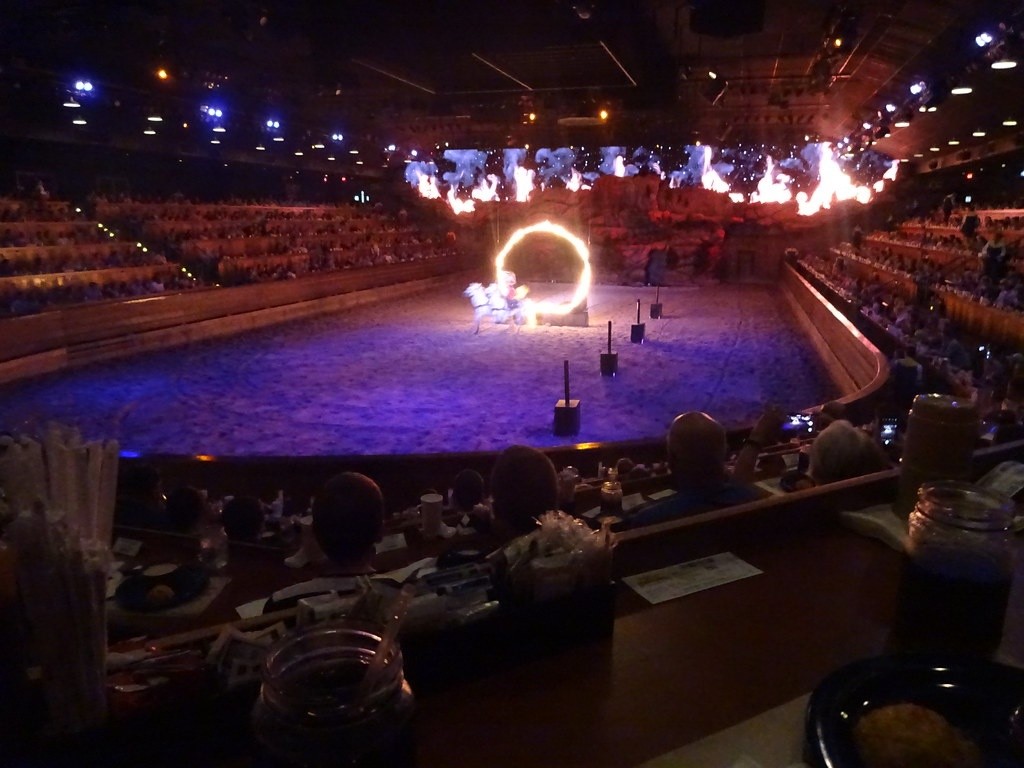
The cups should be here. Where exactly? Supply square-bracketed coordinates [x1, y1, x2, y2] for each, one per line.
[415, 494, 442, 541]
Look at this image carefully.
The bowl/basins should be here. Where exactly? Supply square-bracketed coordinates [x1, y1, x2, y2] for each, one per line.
[803, 652, 1024, 768]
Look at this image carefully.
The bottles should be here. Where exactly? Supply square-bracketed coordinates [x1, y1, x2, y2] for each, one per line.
[252, 629, 415, 768]
[890, 479, 1016, 661]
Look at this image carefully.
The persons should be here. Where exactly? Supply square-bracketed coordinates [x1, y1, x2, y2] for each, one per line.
[0, 393, 1024, 635]
[0, 177, 472, 319]
[803, 190, 1024, 399]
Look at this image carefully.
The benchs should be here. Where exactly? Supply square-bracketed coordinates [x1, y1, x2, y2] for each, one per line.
[0, 201, 1024, 675]
